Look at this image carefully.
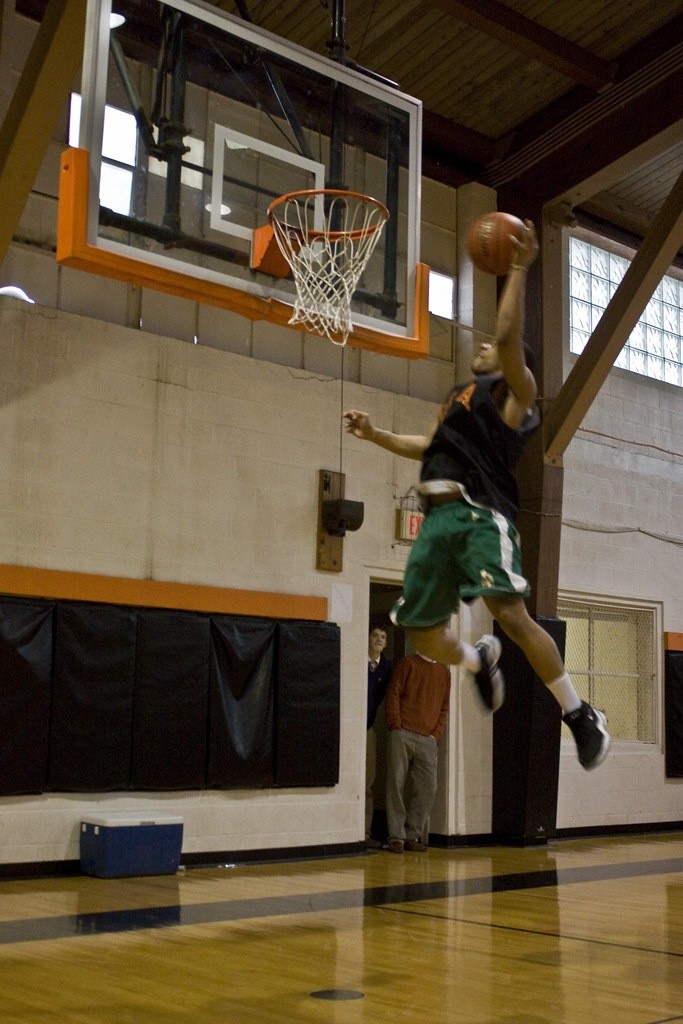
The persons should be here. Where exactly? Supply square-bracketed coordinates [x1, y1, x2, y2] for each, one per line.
[366, 627, 450, 854]
[338, 219, 610, 772]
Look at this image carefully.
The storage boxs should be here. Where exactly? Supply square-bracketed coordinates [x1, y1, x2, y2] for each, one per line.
[80, 813, 184, 877]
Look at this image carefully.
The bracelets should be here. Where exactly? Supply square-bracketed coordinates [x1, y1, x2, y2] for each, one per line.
[510, 262, 528, 275]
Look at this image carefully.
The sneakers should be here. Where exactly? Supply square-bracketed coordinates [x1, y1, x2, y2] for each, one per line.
[471, 635, 504, 712]
[562, 701, 610, 769]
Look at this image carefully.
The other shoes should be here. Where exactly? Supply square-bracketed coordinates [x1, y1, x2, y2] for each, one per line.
[406, 839, 427, 851]
[390, 840, 403, 853]
[368, 839, 383, 848]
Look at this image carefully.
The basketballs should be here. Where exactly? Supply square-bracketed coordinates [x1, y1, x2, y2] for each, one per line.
[465, 209, 530, 278]
